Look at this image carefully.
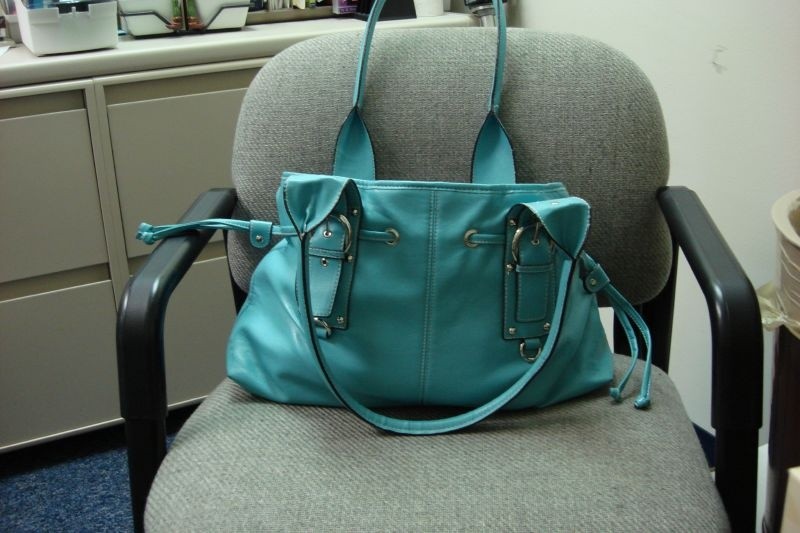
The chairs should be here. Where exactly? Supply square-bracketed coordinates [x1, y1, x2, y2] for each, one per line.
[110, 24, 769, 533]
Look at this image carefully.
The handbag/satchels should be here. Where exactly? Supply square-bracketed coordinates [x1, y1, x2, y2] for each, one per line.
[136, 0, 651, 437]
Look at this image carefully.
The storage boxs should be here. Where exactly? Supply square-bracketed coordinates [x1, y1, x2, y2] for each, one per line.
[14, 0, 121, 57]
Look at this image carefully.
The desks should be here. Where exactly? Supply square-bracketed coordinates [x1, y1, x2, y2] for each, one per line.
[762, 332, 800, 533]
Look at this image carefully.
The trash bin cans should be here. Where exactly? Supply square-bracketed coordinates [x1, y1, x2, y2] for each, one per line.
[759, 187, 800, 532]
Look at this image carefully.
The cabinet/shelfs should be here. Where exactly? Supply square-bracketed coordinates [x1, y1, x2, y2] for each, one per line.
[0, 53, 269, 476]
